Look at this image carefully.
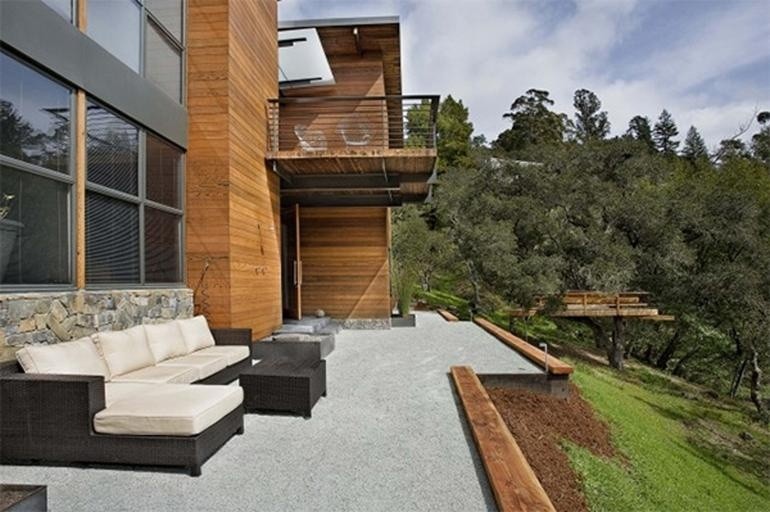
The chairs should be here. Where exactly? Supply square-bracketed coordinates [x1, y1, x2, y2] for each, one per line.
[334, 113, 377, 149]
[293, 120, 328, 152]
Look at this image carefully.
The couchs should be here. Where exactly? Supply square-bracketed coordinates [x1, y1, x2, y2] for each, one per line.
[0, 327, 253, 476]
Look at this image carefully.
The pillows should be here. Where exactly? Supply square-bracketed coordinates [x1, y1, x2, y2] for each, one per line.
[15, 337, 111, 383]
[175, 315, 215, 355]
[90, 325, 154, 379]
[143, 320, 186, 365]
